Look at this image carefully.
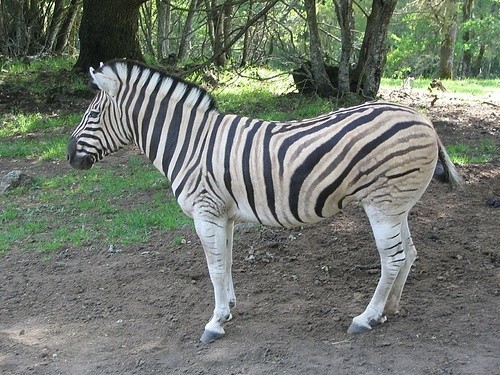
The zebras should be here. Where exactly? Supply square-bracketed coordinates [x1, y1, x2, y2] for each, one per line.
[65, 57, 462, 345]
